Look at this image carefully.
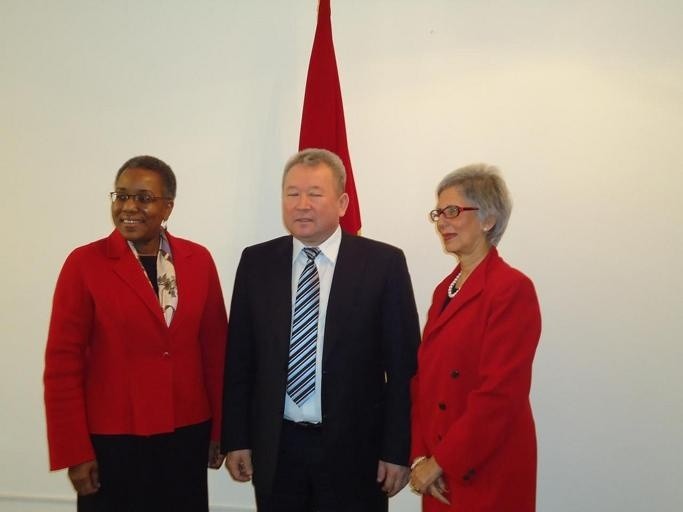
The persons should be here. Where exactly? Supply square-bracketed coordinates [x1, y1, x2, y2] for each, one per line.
[221, 147, 421, 512]
[409, 162, 542, 512]
[43, 155, 229, 512]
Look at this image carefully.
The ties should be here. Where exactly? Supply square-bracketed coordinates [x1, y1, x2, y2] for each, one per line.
[286, 247, 321, 408]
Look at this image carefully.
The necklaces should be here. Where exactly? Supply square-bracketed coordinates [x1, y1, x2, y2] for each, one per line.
[448, 271, 460, 298]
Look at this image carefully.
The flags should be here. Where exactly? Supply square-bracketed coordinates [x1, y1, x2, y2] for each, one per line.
[299, 1, 362, 237]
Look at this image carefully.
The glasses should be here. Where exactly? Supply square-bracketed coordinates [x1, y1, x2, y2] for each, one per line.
[430, 205, 480, 221]
[108, 191, 173, 204]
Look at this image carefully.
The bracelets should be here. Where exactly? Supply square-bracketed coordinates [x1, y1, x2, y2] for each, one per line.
[410, 455, 425, 470]
